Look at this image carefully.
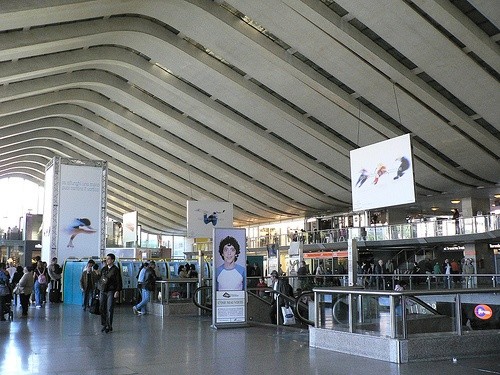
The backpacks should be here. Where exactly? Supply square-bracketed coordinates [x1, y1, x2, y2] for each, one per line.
[36, 268, 46, 283]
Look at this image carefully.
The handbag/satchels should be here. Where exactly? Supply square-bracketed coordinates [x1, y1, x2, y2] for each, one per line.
[281, 306, 296, 325]
[89, 297, 101, 315]
[13, 283, 21, 295]
[0, 285, 9, 297]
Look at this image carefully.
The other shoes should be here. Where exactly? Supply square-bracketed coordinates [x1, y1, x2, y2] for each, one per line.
[133, 306, 137, 316]
[101, 326, 112, 333]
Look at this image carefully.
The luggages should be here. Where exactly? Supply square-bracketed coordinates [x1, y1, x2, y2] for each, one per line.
[49, 279, 61, 303]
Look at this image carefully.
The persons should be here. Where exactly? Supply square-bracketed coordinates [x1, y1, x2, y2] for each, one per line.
[246, 260, 252, 287]
[250, 262, 261, 276]
[80, 253, 122, 333]
[452, 208, 460, 236]
[132, 260, 160, 315]
[411, 261, 421, 286]
[425, 258, 475, 289]
[177, 263, 198, 299]
[316, 258, 393, 288]
[296, 260, 307, 291]
[293, 231, 298, 242]
[215, 235, 245, 290]
[0, 256, 62, 321]
[394, 279, 417, 338]
[269, 264, 294, 327]
[255, 277, 268, 298]
